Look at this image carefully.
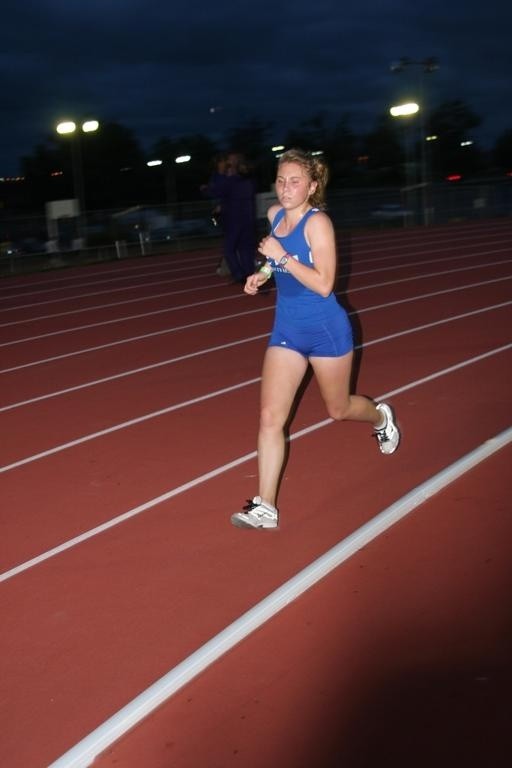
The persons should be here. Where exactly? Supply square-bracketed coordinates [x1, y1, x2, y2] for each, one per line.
[213, 157, 237, 278]
[202, 153, 256, 283]
[229, 149, 400, 532]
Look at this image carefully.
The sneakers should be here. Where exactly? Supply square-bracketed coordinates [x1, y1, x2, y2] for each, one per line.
[374, 403, 400, 455]
[231, 495, 278, 529]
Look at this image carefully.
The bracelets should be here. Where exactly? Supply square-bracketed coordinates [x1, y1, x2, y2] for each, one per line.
[259, 266, 272, 279]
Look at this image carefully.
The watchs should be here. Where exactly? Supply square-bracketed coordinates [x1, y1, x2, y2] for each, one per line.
[278, 252, 292, 267]
